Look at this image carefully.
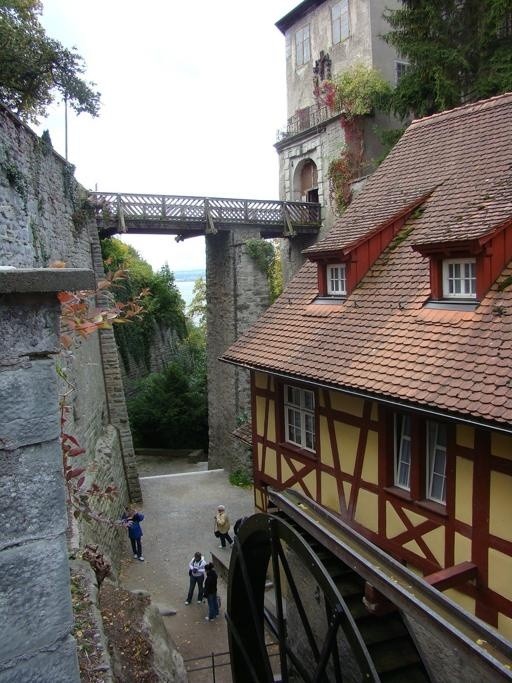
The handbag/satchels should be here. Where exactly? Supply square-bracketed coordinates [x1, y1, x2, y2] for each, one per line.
[214, 530, 222, 538]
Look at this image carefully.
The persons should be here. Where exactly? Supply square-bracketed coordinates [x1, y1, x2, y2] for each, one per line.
[203, 561, 220, 621]
[213, 504, 235, 549]
[184, 551, 207, 605]
[121, 505, 145, 561]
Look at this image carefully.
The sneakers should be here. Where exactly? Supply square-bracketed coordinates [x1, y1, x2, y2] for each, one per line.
[229, 540, 235, 547]
[205, 616, 215, 622]
[138, 557, 145, 561]
[218, 545, 226, 549]
[133, 554, 138, 559]
[197, 600, 202, 604]
[185, 601, 189, 605]
